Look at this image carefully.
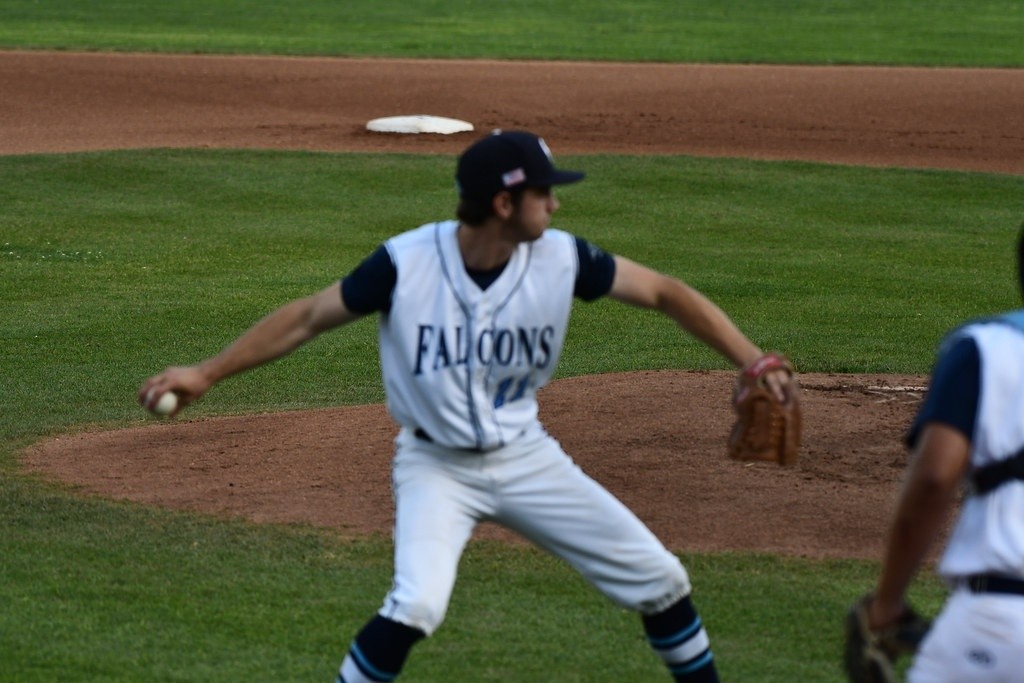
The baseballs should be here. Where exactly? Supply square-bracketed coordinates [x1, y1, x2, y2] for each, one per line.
[143, 385, 178, 415]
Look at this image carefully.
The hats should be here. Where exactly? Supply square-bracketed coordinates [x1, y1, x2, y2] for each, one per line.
[456, 129, 586, 188]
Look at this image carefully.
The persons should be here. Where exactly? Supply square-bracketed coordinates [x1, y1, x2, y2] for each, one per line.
[842, 224, 1024, 683]
[138, 126, 802, 683]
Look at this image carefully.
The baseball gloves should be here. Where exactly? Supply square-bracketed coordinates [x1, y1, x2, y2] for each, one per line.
[841, 587, 932, 683]
[729, 348, 802, 464]
